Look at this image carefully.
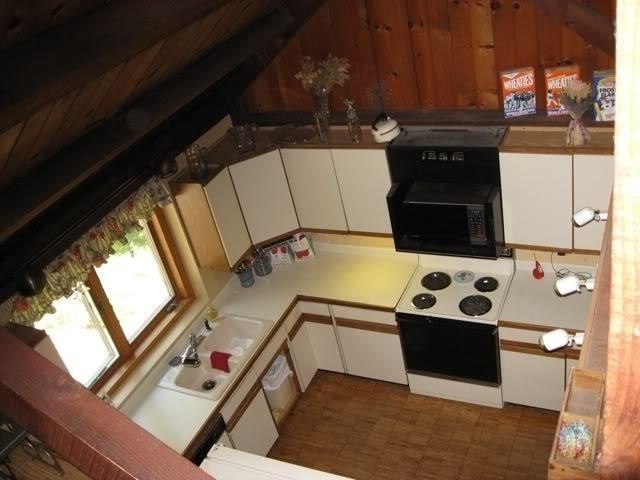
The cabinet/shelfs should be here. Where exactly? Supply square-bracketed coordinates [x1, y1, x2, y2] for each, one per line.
[170, 122, 614, 271]
[170, 242, 599, 467]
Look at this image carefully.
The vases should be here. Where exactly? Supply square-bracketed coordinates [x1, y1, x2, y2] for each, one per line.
[550, 77, 593, 145]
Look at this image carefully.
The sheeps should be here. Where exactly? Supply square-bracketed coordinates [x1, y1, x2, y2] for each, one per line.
[189, 331, 197, 350]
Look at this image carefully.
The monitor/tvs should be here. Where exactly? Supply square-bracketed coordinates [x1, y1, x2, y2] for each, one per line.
[592, 67, 615, 123]
[499, 64, 537, 120]
[545, 62, 581, 118]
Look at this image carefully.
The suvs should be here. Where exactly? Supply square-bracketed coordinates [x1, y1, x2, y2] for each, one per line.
[234, 259, 255, 288]
[252, 253, 273, 276]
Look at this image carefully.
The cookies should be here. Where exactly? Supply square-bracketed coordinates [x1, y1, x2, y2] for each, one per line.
[385, 185, 505, 260]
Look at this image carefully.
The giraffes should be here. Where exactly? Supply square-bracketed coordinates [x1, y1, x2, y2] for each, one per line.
[396, 266, 513, 323]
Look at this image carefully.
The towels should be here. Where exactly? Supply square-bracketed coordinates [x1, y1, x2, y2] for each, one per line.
[261, 356, 296, 413]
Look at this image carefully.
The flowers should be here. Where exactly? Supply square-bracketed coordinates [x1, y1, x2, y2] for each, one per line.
[550, 77, 593, 145]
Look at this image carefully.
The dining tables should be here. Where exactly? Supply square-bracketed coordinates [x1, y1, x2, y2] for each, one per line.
[345, 99, 363, 145]
[300, 52, 351, 145]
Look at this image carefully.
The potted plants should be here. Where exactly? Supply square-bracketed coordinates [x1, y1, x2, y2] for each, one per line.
[300, 52, 351, 145]
[345, 99, 363, 145]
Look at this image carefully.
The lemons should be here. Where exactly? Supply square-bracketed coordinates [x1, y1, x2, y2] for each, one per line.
[370, 105, 401, 145]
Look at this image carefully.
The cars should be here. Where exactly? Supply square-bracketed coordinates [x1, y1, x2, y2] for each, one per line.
[185, 143, 209, 181]
[229, 120, 260, 157]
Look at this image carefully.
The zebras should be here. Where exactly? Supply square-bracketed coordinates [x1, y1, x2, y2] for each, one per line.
[395, 305, 507, 411]
